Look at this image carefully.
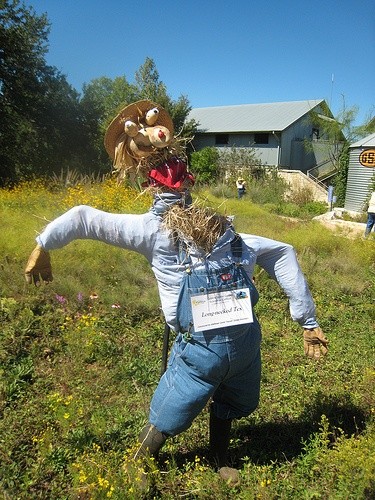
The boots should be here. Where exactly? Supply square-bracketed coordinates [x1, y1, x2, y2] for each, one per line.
[209, 413, 241, 486]
[364, 228, 371, 237]
[130, 425, 168, 495]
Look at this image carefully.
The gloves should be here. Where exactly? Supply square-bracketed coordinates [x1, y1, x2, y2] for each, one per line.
[25, 246, 53, 287]
[303, 324, 329, 360]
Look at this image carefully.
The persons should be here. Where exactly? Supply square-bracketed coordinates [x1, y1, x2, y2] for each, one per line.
[364, 191, 375, 239]
[236, 177, 247, 198]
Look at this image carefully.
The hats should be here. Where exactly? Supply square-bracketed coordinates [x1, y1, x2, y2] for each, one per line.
[103, 100, 174, 160]
[237, 177, 245, 181]
